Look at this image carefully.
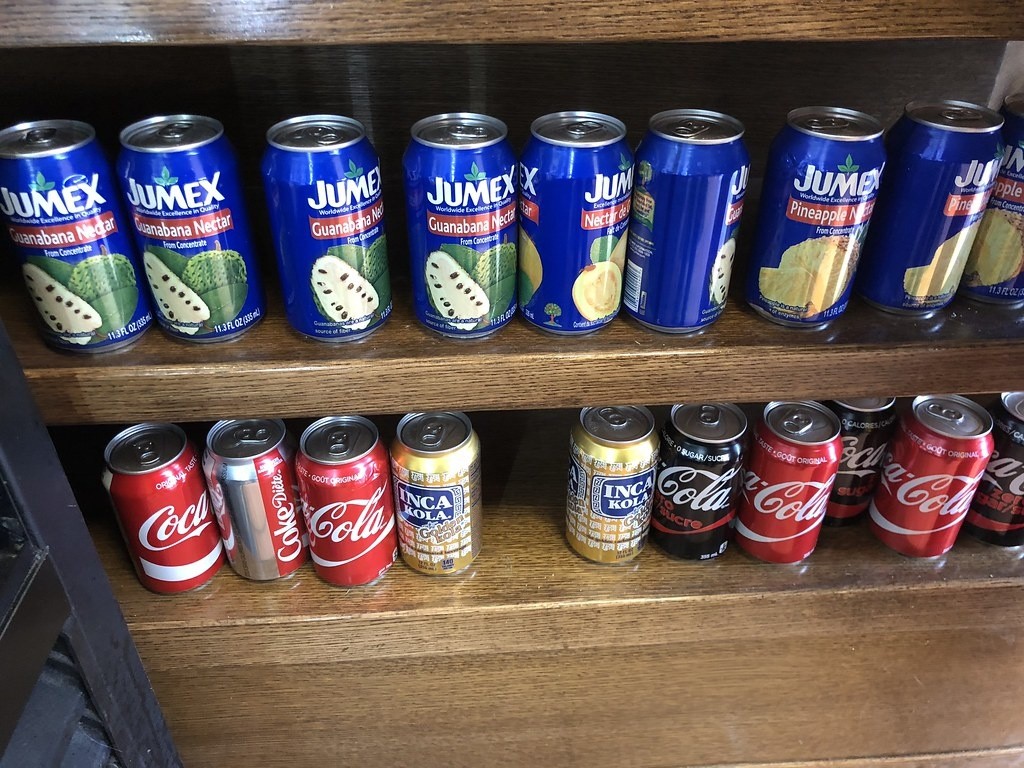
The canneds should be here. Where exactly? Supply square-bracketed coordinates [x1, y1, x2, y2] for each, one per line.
[0, 94, 1024, 598]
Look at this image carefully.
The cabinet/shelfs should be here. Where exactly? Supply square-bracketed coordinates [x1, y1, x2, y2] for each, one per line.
[0, 1, 1024, 768]
